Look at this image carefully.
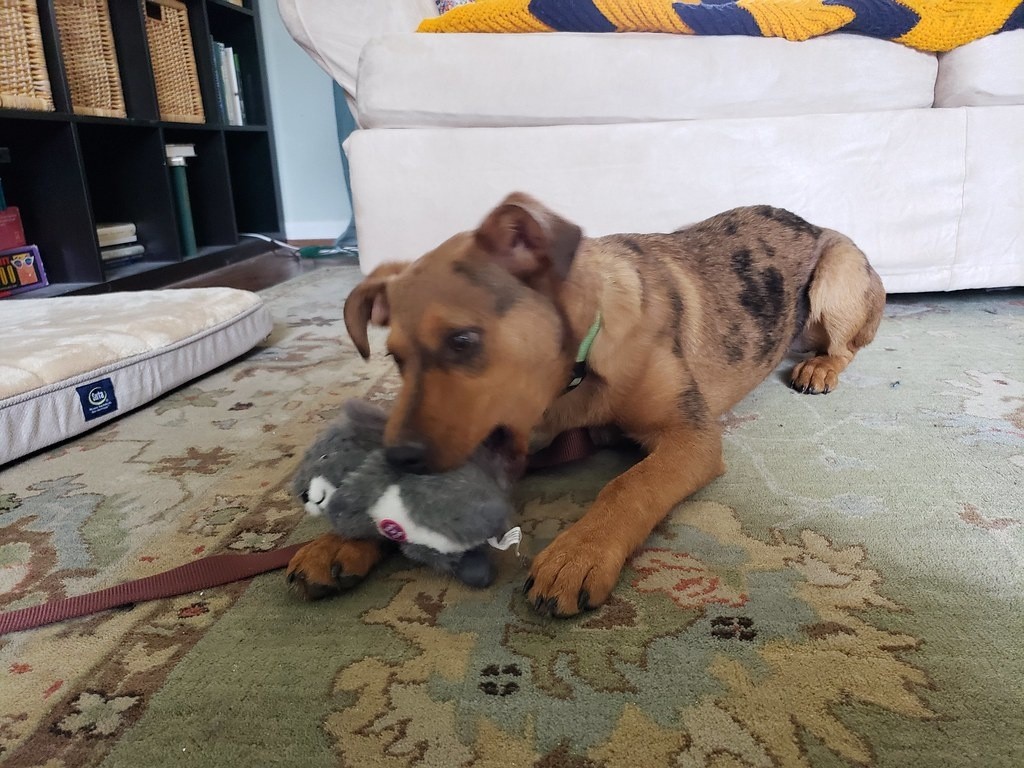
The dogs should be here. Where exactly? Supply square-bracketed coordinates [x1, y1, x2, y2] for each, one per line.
[285, 191, 887, 620]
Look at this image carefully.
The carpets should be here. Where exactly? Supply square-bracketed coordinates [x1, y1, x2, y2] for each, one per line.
[0, 263, 1024, 768]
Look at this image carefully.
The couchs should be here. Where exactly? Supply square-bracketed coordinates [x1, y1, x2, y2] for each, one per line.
[278, 0, 1024, 293]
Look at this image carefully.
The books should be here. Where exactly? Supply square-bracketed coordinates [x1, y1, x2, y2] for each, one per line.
[209, 35, 247, 126]
[96, 222, 145, 269]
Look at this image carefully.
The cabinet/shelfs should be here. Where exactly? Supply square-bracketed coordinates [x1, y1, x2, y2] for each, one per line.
[0, 0, 287, 300]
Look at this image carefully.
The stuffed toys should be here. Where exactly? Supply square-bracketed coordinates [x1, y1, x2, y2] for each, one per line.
[289, 402, 516, 589]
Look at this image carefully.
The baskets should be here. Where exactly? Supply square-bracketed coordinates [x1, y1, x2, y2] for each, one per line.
[0, 0, 56, 113]
[142, 0, 207, 123]
[55, 0, 127, 118]
